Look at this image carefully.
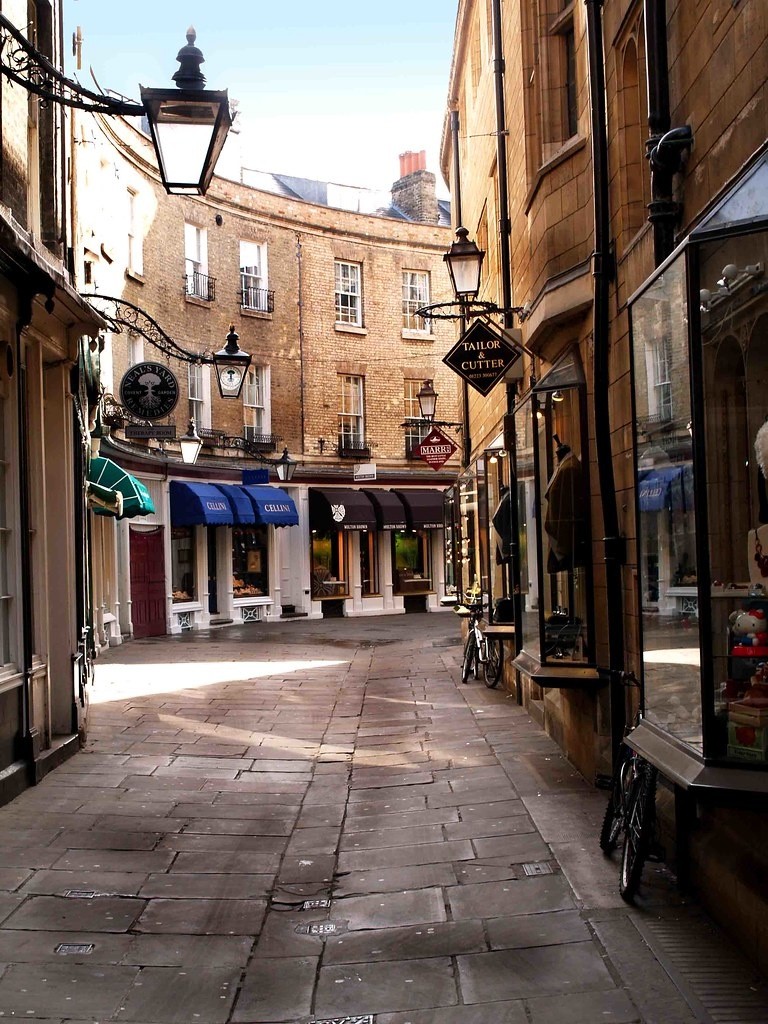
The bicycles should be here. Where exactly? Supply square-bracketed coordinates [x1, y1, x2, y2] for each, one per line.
[451, 591, 504, 688]
[596, 665, 656, 898]
